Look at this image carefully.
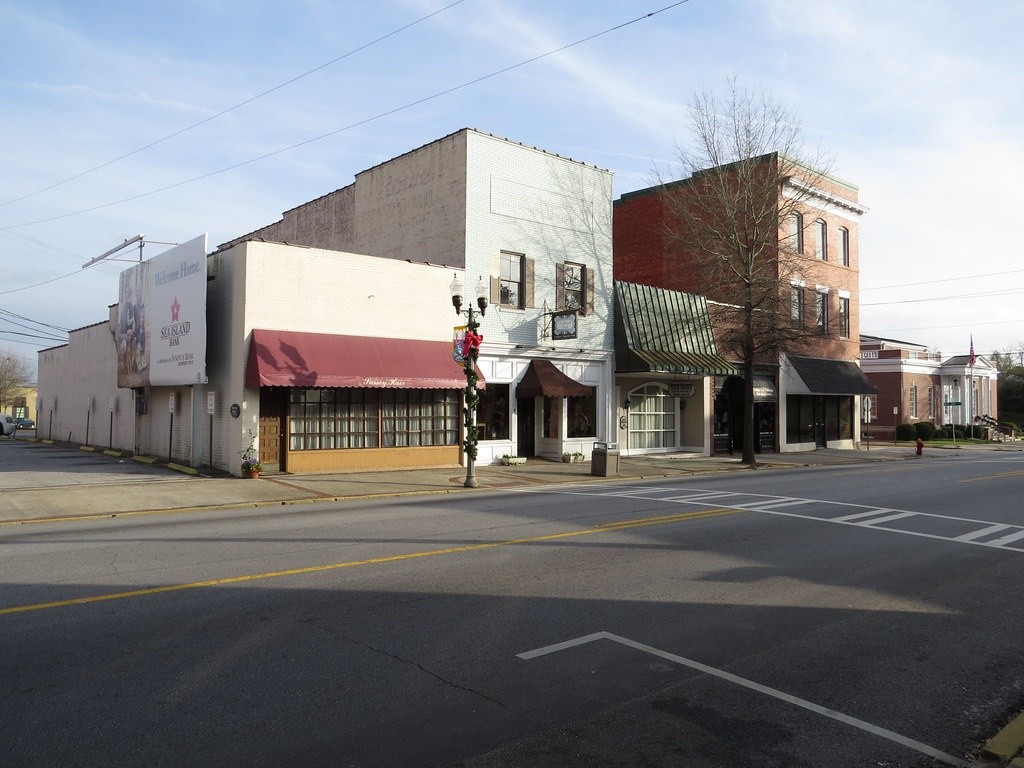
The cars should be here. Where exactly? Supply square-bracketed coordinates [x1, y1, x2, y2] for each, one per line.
[0, 413, 17, 436]
[13, 417, 35, 430]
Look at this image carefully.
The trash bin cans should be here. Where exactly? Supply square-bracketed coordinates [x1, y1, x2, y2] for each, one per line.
[592, 441, 620, 477]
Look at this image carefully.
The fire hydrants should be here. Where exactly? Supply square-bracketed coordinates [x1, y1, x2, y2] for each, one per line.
[915, 437, 924, 455]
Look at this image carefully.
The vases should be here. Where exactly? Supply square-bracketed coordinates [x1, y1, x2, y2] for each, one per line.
[241, 469, 260, 479]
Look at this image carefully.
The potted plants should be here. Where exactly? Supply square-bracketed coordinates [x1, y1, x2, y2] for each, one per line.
[562, 451, 585, 463]
[501, 454, 527, 466]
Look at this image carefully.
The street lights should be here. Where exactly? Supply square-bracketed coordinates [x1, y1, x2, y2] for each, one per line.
[449, 271, 488, 488]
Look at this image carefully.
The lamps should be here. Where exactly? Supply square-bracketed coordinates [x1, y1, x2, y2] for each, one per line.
[623, 398, 631, 409]
[953, 379, 958, 386]
[973, 381, 975, 387]
[680, 400, 686, 409]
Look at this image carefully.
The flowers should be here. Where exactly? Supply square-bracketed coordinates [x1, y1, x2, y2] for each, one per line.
[236, 428, 263, 472]
